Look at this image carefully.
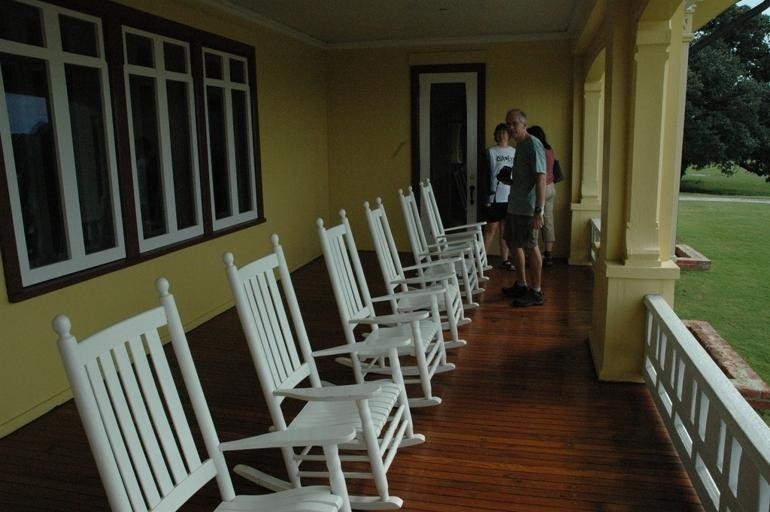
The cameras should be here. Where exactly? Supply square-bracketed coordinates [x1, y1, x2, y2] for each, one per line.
[499, 165, 512, 178]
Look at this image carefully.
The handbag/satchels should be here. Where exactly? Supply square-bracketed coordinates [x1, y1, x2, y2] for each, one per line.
[552, 159, 565, 185]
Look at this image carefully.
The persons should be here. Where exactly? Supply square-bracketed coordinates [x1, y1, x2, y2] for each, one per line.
[482, 108, 564, 306]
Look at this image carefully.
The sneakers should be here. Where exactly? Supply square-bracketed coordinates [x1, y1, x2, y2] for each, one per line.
[514, 286, 544, 308]
[541, 250, 553, 269]
[501, 280, 528, 297]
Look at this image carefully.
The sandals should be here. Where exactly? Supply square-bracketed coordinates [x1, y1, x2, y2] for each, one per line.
[500, 258, 517, 270]
